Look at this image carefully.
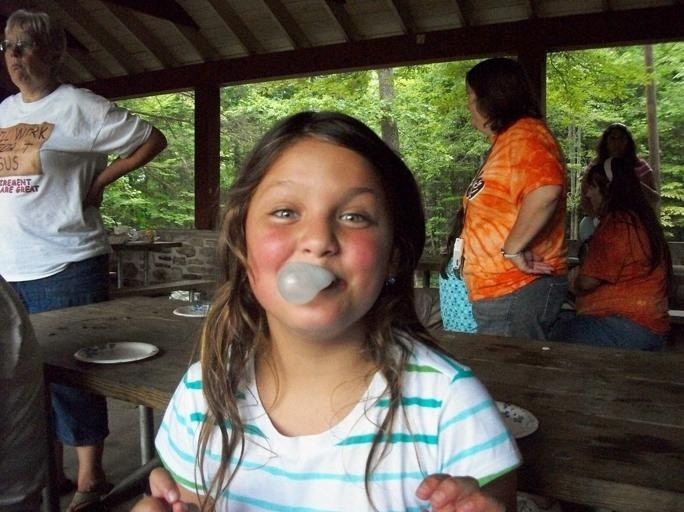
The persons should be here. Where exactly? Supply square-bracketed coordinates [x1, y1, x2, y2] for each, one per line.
[130, 110, 527, 512]
[577, 122, 661, 242]
[436, 208, 479, 336]
[549, 154, 673, 351]
[0, 5, 170, 512]
[457, 55, 570, 343]
[1, 273, 51, 512]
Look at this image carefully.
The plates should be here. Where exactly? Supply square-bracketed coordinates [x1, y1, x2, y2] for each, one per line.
[73, 341, 162, 364]
[495, 402, 540, 440]
[172, 304, 209, 319]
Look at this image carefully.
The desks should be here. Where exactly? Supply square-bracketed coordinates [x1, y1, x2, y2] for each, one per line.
[24, 296, 682, 509]
[108, 239, 183, 288]
[412, 257, 682, 312]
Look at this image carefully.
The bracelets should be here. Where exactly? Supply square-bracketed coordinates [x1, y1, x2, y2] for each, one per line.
[499, 248, 523, 259]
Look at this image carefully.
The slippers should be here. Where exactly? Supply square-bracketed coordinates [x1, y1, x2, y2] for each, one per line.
[59, 479, 114, 512]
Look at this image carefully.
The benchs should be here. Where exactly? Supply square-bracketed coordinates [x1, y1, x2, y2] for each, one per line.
[108, 277, 218, 302]
